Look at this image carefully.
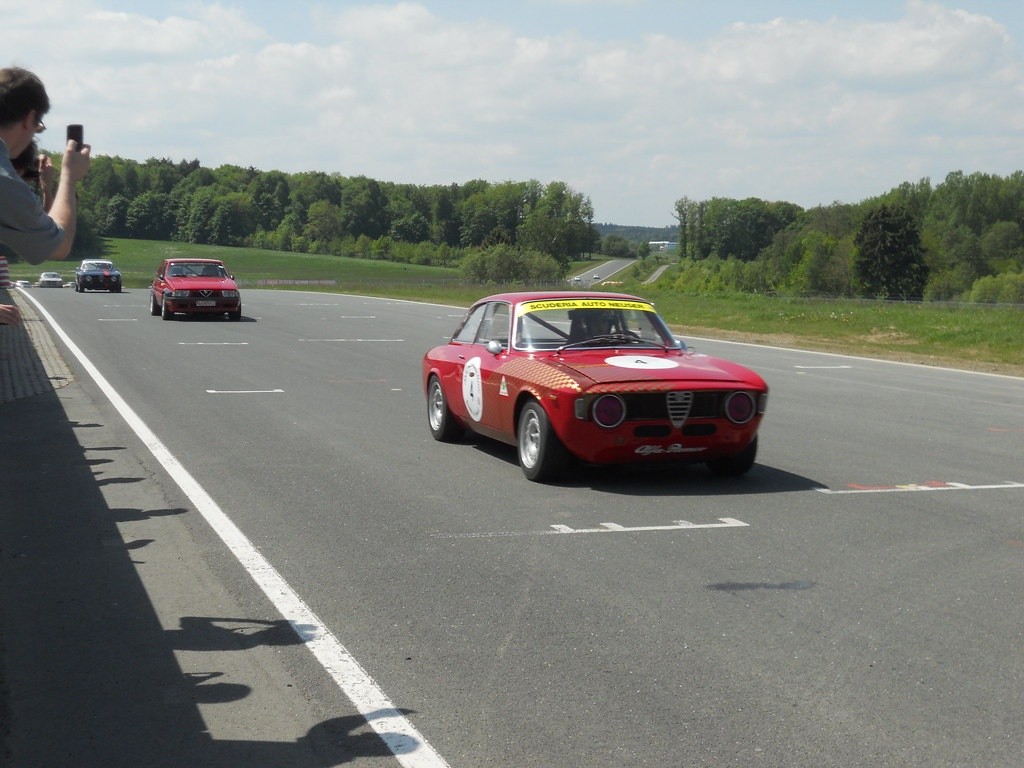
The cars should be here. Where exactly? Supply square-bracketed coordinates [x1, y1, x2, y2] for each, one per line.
[76, 259, 122, 293]
[11, 280, 75, 289]
[424, 289, 770, 484]
[594, 274, 600, 279]
[38, 272, 63, 289]
[575, 276, 581, 280]
[147, 257, 242, 321]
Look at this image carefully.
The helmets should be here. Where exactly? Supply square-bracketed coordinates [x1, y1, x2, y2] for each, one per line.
[581, 310, 616, 334]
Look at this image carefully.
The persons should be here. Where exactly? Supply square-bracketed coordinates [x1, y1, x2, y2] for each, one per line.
[581, 310, 614, 343]
[0, 67, 89, 328]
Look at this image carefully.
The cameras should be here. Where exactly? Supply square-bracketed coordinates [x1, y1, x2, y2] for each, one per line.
[22, 158, 39, 180]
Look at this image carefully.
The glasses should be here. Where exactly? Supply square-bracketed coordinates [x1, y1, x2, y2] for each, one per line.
[36, 117, 46, 133]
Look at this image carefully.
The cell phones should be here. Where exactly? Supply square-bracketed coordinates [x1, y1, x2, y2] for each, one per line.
[66, 124, 84, 152]
